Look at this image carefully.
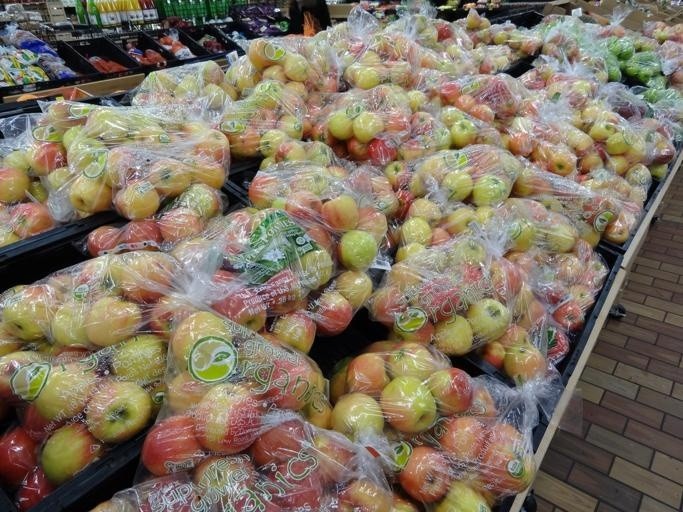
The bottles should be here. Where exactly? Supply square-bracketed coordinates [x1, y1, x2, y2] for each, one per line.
[156, 0, 248, 20]
[87, 0, 101, 26]
[76, 0, 88, 25]
[95, 0, 159, 28]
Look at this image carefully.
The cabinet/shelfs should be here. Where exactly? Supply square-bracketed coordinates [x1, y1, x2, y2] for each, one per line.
[1, 0, 683, 512]
[0, 0, 292, 104]
[0, 23, 273, 110]
